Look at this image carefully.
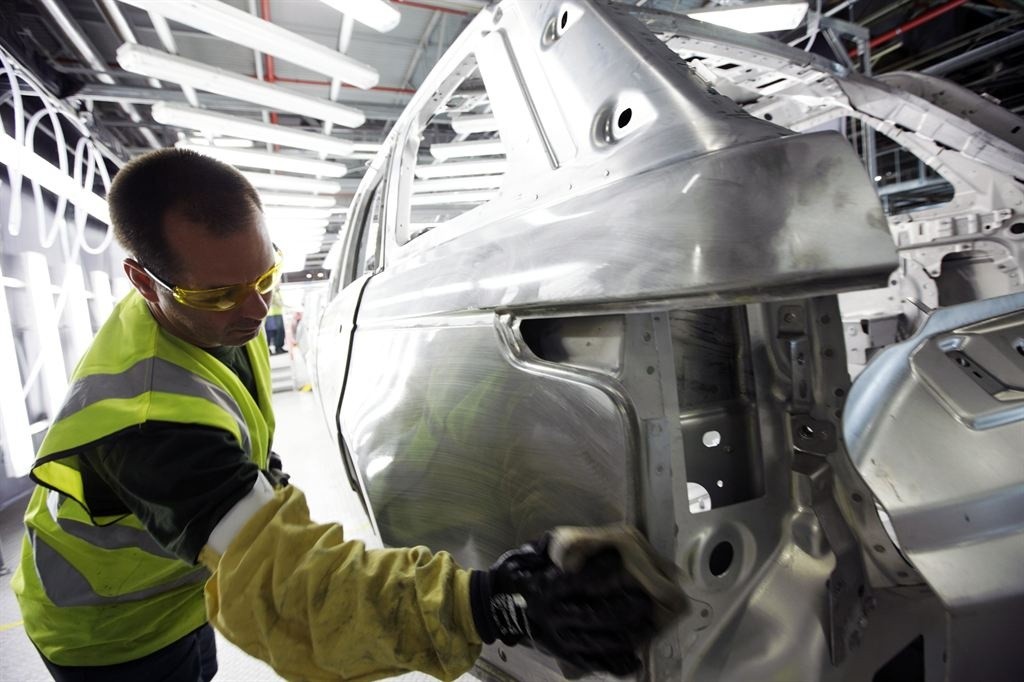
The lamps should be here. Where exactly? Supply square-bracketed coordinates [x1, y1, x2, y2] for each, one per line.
[411, 114, 507, 205]
[114, 0, 400, 266]
[684, 0, 809, 34]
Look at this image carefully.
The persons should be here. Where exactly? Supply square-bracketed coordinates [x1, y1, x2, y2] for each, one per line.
[8, 148, 671, 682]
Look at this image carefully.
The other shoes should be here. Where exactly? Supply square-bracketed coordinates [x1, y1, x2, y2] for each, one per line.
[275, 347, 287, 353]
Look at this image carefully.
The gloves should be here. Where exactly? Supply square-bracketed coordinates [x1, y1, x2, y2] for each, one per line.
[469, 535, 658, 679]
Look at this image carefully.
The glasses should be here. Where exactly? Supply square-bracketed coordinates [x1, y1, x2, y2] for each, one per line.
[141, 244, 282, 312]
